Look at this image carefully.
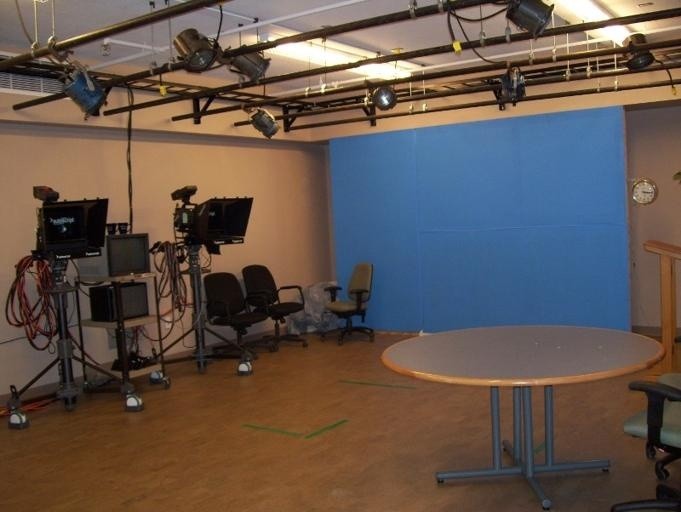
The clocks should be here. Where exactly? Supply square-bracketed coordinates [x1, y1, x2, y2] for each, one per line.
[631, 178, 657, 205]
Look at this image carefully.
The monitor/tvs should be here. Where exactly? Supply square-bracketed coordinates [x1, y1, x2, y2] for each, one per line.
[76, 233, 150, 276]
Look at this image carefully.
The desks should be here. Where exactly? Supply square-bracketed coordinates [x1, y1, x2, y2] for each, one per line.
[378, 324, 667, 511]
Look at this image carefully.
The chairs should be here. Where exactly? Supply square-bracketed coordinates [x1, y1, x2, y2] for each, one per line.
[319, 262, 376, 345]
[202, 263, 310, 361]
[610, 371, 681, 511]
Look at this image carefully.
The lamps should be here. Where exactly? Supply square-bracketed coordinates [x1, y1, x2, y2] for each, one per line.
[503, 0, 657, 77]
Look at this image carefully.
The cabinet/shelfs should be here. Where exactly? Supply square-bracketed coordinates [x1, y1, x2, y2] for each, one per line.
[75, 272, 171, 399]
